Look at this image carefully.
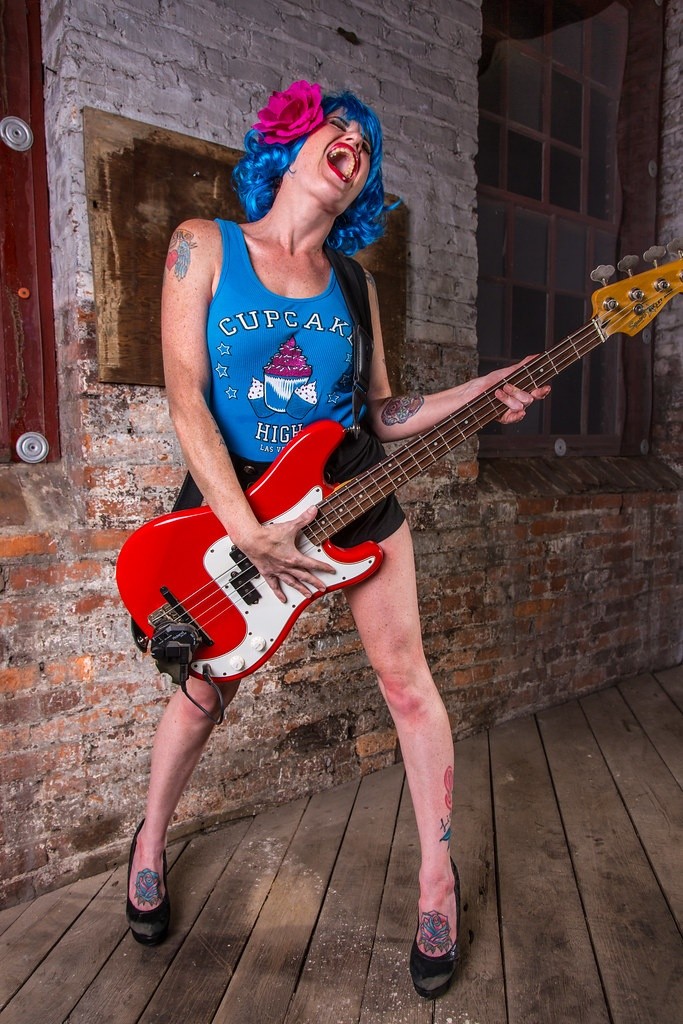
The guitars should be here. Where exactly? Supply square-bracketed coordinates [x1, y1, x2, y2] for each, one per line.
[115, 259, 683, 685]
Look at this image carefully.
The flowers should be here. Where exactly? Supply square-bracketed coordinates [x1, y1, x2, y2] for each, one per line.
[251, 79, 325, 144]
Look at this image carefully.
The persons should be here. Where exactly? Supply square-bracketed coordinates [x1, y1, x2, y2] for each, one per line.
[125, 87, 553, 1004]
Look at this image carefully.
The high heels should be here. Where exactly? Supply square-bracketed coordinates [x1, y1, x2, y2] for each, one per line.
[409, 856, 462, 999]
[124, 818, 170, 946]
